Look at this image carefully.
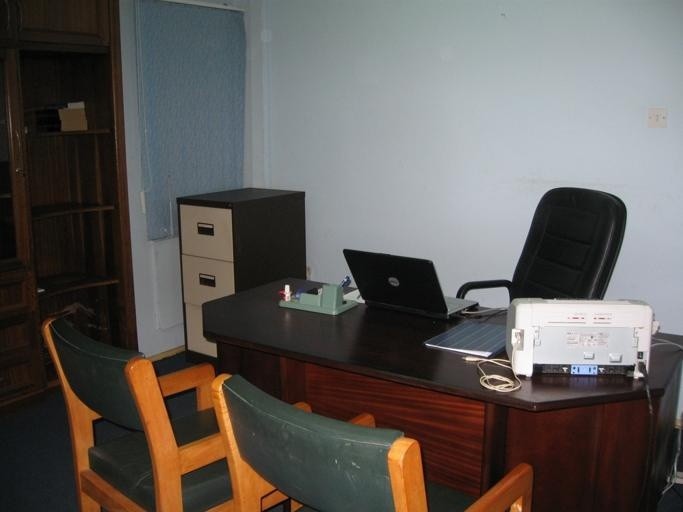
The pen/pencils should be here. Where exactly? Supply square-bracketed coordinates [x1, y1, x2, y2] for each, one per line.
[339, 276, 351, 290]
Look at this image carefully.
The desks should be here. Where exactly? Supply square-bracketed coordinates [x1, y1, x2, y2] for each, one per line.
[201, 277, 682, 510]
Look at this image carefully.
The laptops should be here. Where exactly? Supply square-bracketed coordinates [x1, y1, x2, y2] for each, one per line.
[343, 248, 479, 321]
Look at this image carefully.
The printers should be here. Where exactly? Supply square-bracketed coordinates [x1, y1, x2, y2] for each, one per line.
[504, 297, 655, 382]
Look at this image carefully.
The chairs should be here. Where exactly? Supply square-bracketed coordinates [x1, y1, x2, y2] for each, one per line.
[456, 187, 625, 321]
[41, 308, 312, 512]
[211, 373, 534, 511]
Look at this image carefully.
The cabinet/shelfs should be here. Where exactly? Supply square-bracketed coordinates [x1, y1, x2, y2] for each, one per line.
[176, 187, 306, 367]
[0, 0, 138, 405]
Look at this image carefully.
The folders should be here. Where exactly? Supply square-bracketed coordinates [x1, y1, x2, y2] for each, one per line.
[422, 318, 506, 359]
